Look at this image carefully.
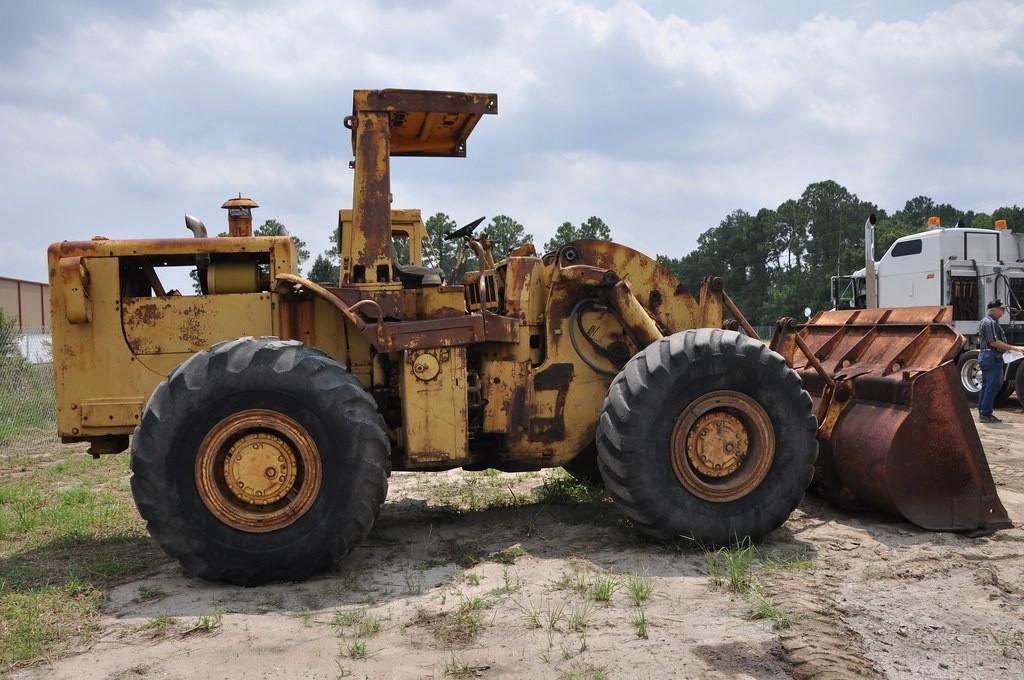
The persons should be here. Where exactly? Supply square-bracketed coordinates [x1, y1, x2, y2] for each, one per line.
[975, 299, 1023, 423]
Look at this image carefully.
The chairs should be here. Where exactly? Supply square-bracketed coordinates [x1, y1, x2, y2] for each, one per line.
[390, 242, 444, 284]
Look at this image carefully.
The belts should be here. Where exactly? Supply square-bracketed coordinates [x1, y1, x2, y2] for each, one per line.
[980, 348, 1002, 352]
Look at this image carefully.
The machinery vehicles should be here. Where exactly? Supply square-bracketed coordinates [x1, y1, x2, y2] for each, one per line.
[44, 87, 1015, 589]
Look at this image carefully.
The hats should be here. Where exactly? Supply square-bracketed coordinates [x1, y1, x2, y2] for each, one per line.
[988, 299, 1008, 308]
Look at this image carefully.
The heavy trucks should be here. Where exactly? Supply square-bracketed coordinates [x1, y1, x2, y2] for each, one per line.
[830, 210, 1024, 414]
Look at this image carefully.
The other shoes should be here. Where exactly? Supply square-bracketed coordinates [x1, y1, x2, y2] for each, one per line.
[979, 413, 1002, 423]
